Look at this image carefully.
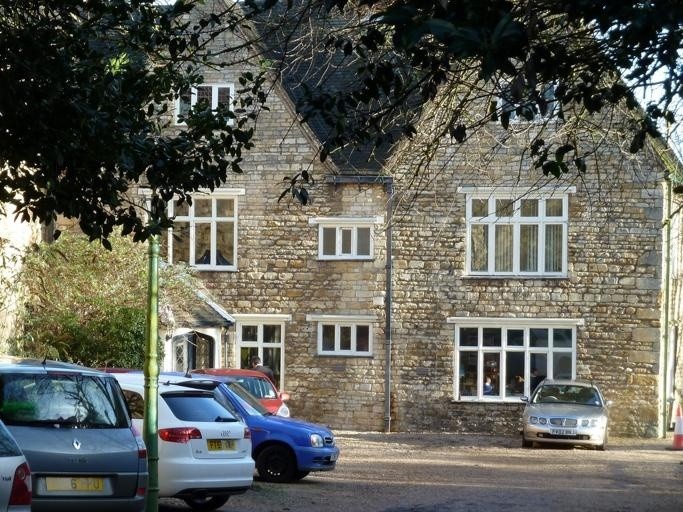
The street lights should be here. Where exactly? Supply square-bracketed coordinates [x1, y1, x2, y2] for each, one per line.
[135, 166, 169, 512]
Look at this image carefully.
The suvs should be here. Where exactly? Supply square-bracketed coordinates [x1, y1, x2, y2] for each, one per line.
[1, 353, 149, 511]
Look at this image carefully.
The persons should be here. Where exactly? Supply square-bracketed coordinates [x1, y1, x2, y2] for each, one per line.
[483, 375, 496, 395]
[250, 355, 276, 387]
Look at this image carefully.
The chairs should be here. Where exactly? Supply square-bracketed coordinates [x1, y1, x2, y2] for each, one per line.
[544, 388, 592, 403]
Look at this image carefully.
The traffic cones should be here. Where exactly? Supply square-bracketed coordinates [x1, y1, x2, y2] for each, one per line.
[665, 403, 682, 451]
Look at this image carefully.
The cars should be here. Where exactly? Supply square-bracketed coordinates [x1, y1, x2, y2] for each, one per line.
[1, 421, 33, 511]
[461, 354, 497, 388]
[156, 372, 341, 484]
[107, 372, 257, 511]
[517, 378, 611, 450]
[183, 368, 291, 416]
